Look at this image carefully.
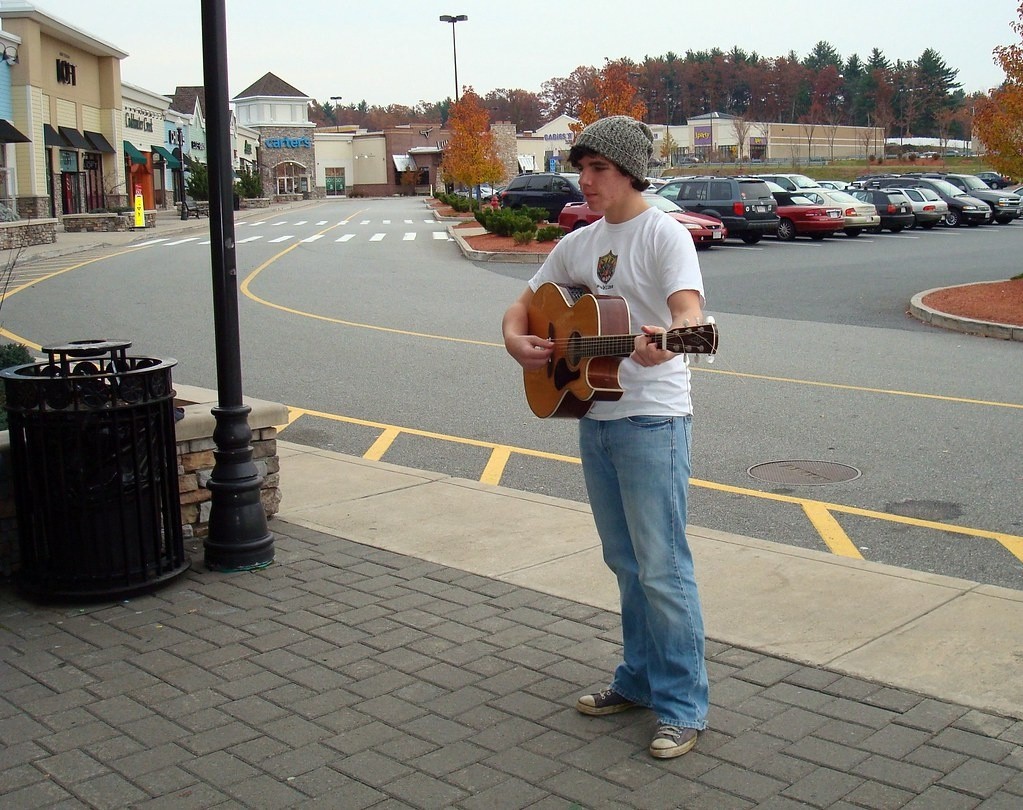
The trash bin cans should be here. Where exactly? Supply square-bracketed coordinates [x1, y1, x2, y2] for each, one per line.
[0, 337, 194, 606]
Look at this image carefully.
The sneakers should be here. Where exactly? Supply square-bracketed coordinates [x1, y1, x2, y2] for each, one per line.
[649, 724, 697, 758]
[576, 689, 639, 715]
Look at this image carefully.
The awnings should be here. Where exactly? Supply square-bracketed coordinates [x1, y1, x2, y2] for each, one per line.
[58, 126, 90, 148]
[0, 119, 33, 143]
[44, 124, 66, 146]
[151, 146, 180, 168]
[124, 141, 146, 163]
[84, 130, 116, 153]
[392, 154, 418, 172]
[518, 156, 539, 171]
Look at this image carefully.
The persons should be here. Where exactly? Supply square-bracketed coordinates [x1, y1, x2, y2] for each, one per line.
[503, 116, 706, 758]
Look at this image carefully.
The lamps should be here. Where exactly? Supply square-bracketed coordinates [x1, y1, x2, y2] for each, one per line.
[0, 41, 20, 65]
[290, 163, 293, 166]
[347, 140, 351, 144]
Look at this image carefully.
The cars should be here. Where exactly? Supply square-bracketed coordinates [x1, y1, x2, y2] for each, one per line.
[814, 173, 900, 196]
[867, 187, 949, 231]
[641, 176, 669, 193]
[453, 183, 508, 201]
[647, 158, 663, 168]
[1010, 187, 1023, 216]
[557, 189, 728, 248]
[973, 172, 1008, 190]
[728, 172, 828, 204]
[835, 189, 914, 234]
[886, 150, 958, 159]
[987, 171, 1012, 182]
[769, 192, 845, 242]
[790, 189, 882, 238]
[679, 157, 700, 163]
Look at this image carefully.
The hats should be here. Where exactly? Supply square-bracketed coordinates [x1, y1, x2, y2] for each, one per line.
[576, 115, 655, 181]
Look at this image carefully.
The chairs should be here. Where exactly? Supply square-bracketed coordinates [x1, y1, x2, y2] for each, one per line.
[688, 188, 697, 199]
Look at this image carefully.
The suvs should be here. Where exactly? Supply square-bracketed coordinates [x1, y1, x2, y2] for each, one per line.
[861, 176, 994, 229]
[652, 177, 780, 246]
[901, 171, 1023, 225]
[499, 172, 587, 224]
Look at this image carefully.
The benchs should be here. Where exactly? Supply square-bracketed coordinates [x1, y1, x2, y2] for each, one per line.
[185, 201, 208, 219]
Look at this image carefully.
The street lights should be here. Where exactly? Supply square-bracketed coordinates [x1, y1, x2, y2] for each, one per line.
[330, 96, 342, 133]
[627, 72, 642, 111]
[174, 117, 189, 221]
[440, 14, 468, 105]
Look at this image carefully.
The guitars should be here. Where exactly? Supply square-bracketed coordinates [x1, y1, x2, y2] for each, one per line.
[523, 283, 720, 421]
